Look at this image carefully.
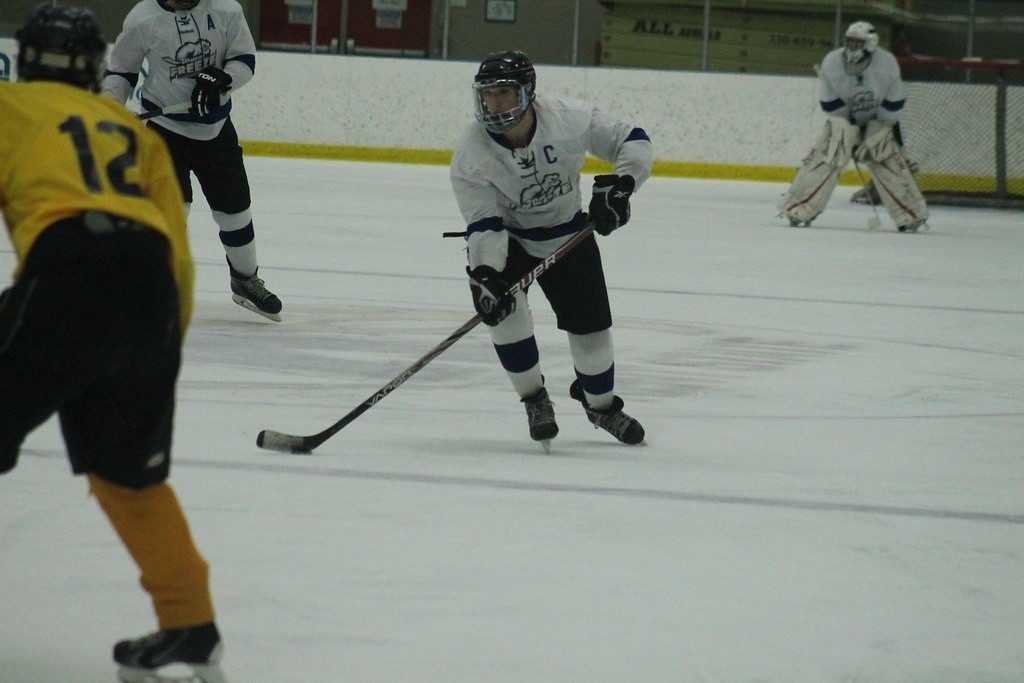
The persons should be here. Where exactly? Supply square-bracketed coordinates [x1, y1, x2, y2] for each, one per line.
[777, 17, 929, 233]
[1, 1, 229, 682]
[98, 0, 282, 324]
[448, 48, 657, 454]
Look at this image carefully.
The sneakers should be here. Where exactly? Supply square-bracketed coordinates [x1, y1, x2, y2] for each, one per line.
[570, 379, 645, 447]
[226, 254, 282, 322]
[520, 374, 559, 455]
[114, 622, 228, 683]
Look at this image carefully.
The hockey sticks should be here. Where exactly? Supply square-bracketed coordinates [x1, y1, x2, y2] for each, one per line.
[253, 224, 593, 451]
[852, 156, 885, 233]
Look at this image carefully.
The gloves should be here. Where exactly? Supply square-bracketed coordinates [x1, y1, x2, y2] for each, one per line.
[586, 174, 635, 236]
[466, 265, 516, 327]
[191, 66, 233, 120]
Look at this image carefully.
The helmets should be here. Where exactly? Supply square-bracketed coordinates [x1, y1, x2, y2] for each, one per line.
[844, 21, 879, 64]
[472, 49, 537, 134]
[14, 0, 109, 94]
